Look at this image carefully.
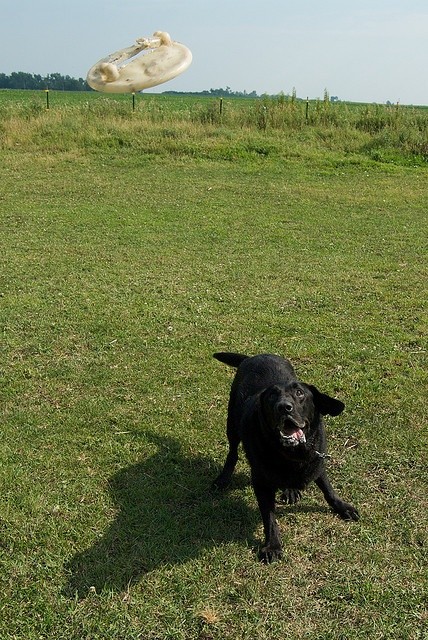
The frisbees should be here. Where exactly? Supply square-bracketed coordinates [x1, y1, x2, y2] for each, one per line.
[87, 31, 192, 94]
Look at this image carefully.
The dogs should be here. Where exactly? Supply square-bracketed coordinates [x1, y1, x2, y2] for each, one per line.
[209, 351, 362, 566]
